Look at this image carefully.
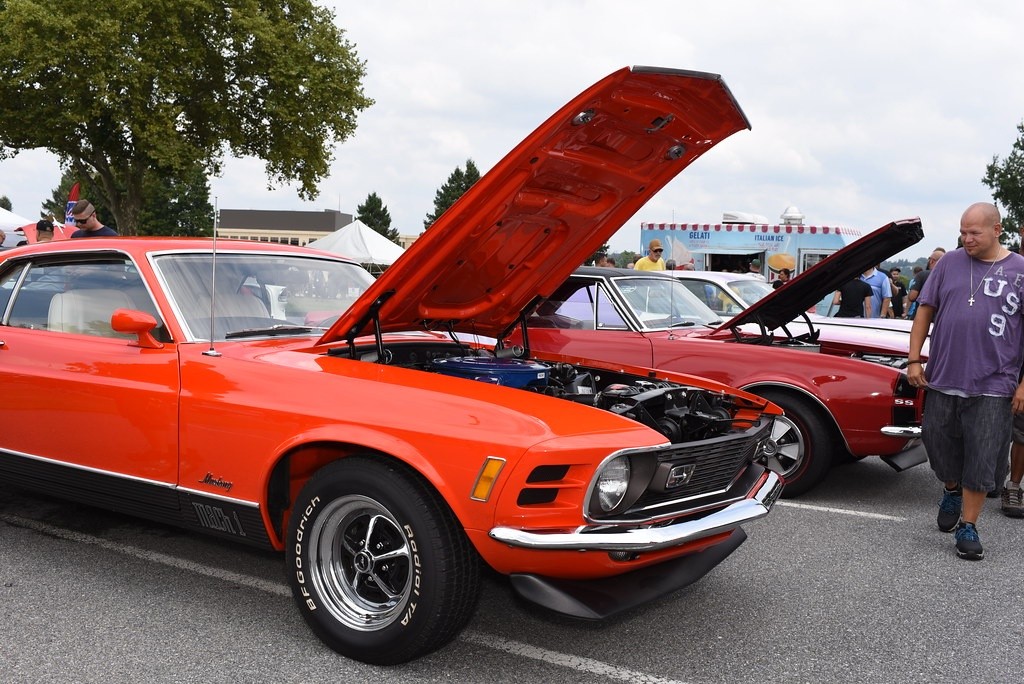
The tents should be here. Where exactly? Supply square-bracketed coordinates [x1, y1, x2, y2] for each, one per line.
[304, 219, 406, 274]
[0, 207, 37, 247]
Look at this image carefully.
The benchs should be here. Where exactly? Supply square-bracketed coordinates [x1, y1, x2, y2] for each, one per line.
[0, 288, 163, 330]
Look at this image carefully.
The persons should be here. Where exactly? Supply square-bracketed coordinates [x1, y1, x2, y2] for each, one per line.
[70, 199, 117, 238]
[0, 229, 6, 248]
[36, 220, 54, 243]
[772, 263, 908, 319]
[595, 239, 766, 311]
[902, 203, 1024, 560]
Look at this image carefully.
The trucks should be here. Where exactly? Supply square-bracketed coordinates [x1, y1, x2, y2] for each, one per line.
[643, 210, 862, 318]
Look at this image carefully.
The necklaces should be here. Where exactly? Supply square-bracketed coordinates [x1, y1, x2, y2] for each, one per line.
[967, 246, 1001, 307]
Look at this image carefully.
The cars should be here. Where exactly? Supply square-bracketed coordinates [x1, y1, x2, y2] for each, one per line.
[0, 64, 784, 669]
[615, 272, 935, 358]
[439, 216, 937, 501]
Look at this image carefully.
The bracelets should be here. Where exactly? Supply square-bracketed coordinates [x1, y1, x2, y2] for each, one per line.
[907, 360, 922, 365]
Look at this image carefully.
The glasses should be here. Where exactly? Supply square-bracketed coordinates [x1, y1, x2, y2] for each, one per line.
[653, 249, 663, 252]
[74, 209, 96, 224]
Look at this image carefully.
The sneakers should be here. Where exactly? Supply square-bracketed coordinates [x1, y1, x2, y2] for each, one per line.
[954, 520, 984, 560]
[986, 464, 1010, 498]
[1001, 481, 1024, 518]
[937, 482, 963, 532]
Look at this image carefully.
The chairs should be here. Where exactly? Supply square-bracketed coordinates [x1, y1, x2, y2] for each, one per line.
[187, 291, 274, 341]
[45, 290, 143, 336]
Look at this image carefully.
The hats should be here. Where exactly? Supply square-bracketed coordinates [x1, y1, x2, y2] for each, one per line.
[890, 267, 901, 273]
[36, 220, 54, 232]
[649, 239, 664, 252]
